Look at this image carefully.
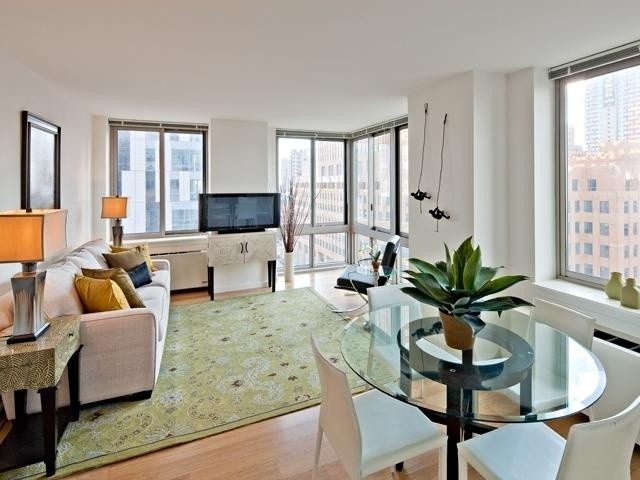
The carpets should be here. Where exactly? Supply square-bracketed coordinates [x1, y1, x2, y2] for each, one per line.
[0, 284, 401, 480]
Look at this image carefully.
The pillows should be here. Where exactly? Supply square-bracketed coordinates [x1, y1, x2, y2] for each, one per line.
[73, 242, 158, 314]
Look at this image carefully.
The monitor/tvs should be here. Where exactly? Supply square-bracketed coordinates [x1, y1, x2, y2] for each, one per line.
[198, 192, 281, 234]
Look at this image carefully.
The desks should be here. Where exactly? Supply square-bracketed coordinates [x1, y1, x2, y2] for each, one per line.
[339, 301, 608, 439]
[0, 314, 80, 471]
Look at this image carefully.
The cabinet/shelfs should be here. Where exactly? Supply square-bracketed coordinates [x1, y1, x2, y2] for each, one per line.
[207, 230, 277, 299]
[110, 233, 208, 294]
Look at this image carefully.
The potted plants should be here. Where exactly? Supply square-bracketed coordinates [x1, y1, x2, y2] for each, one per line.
[398, 236, 536, 349]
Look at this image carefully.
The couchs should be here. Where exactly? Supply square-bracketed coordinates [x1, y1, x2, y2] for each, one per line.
[0, 239, 171, 424]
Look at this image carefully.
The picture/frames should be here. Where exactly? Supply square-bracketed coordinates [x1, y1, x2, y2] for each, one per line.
[19, 109, 61, 210]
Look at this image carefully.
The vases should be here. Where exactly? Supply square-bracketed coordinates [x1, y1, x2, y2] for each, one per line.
[285, 252, 294, 282]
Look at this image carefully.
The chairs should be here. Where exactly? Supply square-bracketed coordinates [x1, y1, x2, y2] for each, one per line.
[531, 297, 598, 353]
[588, 335, 640, 446]
[332, 235, 401, 314]
[311, 336, 448, 479]
[455, 395, 640, 479]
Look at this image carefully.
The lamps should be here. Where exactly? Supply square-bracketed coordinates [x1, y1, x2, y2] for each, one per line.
[101, 195, 128, 246]
[0, 208, 68, 343]
